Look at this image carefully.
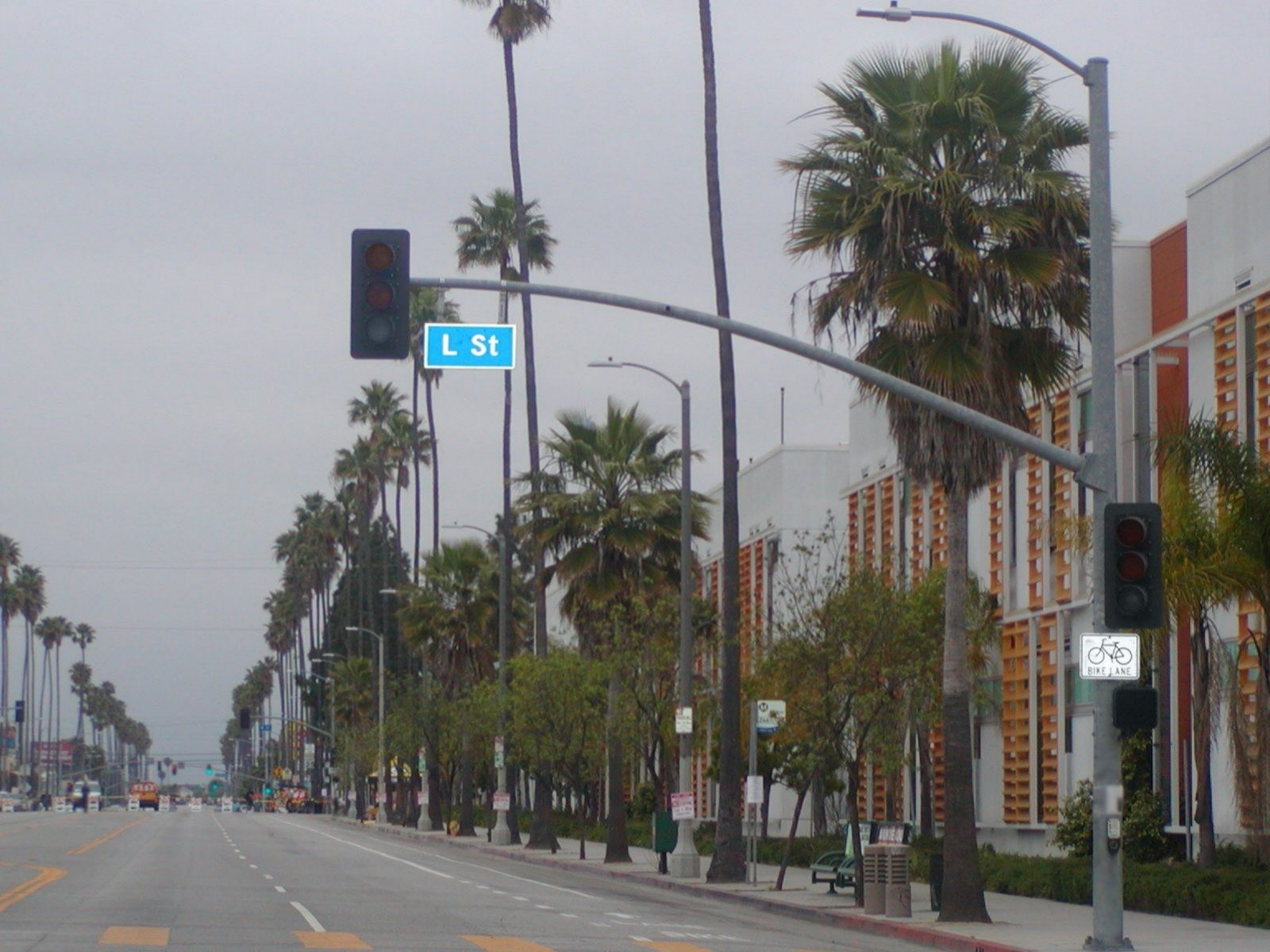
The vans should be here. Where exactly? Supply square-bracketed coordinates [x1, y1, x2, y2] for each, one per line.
[71, 781, 104, 812]
[129, 782, 162, 811]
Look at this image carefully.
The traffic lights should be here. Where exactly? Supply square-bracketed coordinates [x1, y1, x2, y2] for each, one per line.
[172, 764, 177, 776]
[350, 228, 410, 358]
[266, 783, 270, 793]
[1103, 503, 1165, 629]
[207, 764, 211, 773]
[1113, 687, 1158, 730]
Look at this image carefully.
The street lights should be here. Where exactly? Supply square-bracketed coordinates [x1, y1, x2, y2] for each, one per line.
[585, 356, 702, 881]
[346, 626, 388, 825]
[378, 588, 433, 832]
[286, 653, 359, 820]
[853, 0, 1135, 951]
[438, 522, 513, 847]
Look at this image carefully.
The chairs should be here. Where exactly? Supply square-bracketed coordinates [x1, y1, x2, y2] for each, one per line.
[811, 820, 910, 894]
